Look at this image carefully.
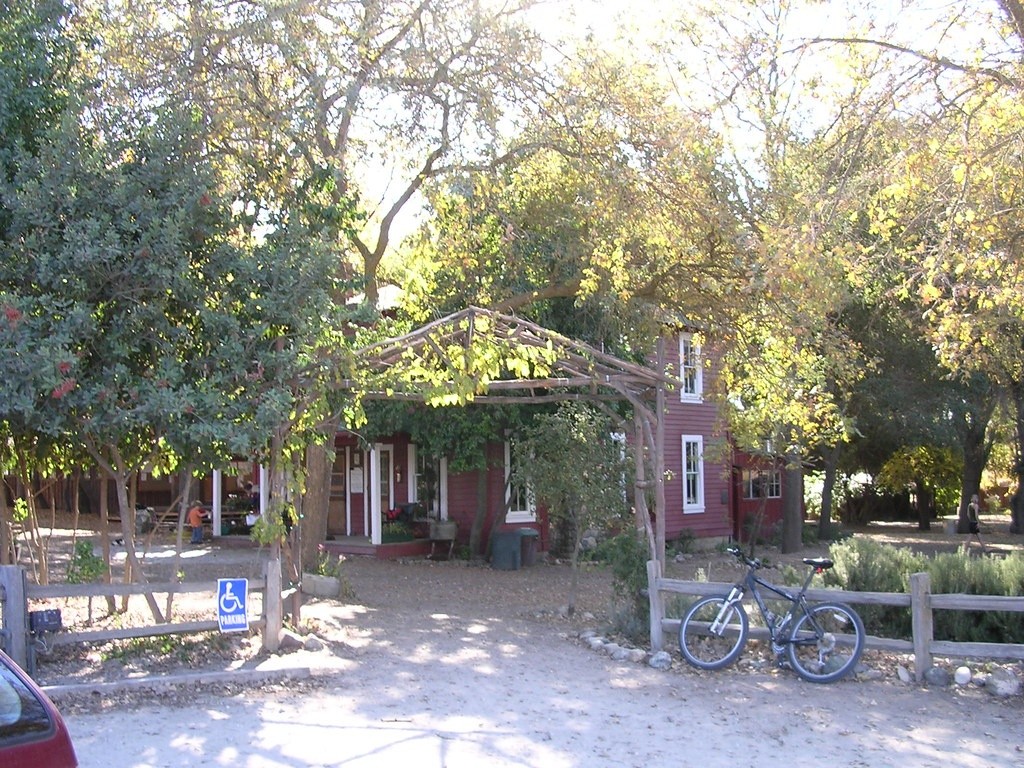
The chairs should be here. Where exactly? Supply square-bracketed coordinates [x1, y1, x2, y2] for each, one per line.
[382, 501, 417, 530]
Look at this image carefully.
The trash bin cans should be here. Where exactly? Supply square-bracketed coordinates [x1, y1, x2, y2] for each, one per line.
[491, 527, 538, 570]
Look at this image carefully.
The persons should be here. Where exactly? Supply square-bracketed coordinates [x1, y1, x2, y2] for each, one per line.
[964, 494, 993, 553]
[190, 500, 208, 544]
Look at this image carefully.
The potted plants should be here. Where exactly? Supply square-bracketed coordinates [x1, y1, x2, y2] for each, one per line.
[382, 523, 414, 544]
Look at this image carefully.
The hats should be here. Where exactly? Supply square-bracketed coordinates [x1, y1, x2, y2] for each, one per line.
[192, 500, 203, 506]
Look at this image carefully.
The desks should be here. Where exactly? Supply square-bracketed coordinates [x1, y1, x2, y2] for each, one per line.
[427, 520, 459, 559]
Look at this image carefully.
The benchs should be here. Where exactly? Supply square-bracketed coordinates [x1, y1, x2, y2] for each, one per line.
[153, 505, 248, 534]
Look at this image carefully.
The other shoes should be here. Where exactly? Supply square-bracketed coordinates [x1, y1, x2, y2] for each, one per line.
[191, 541, 203, 544]
[981, 548, 993, 553]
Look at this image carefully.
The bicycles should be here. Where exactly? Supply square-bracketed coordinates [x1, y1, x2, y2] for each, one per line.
[676, 546, 866, 684]
